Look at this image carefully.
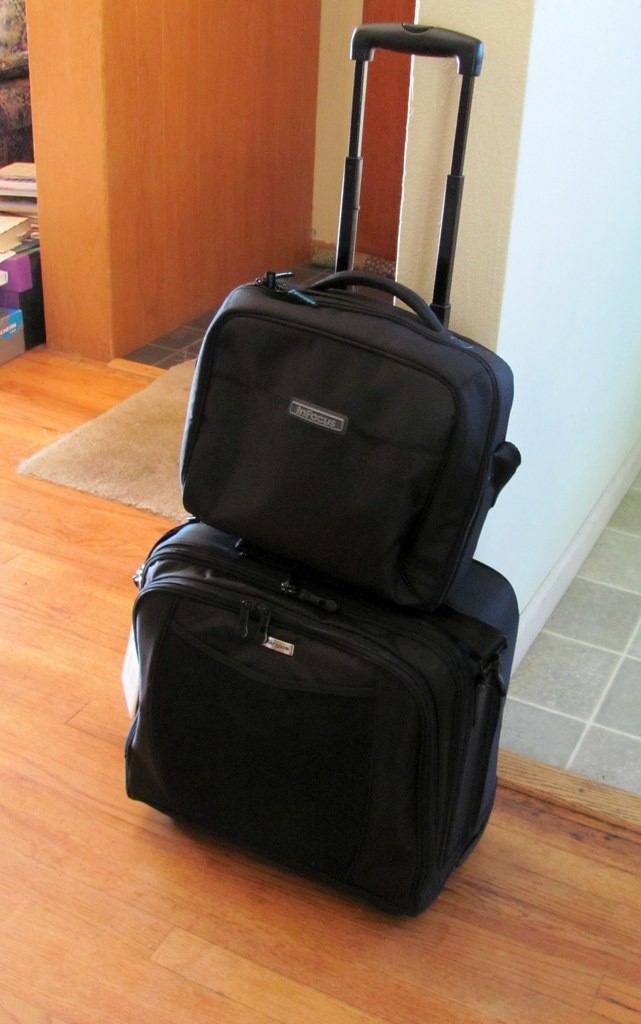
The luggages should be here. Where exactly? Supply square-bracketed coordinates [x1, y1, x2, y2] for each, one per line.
[121, 22, 520, 919]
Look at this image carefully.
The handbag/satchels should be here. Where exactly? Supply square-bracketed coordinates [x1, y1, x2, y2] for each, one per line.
[180, 268, 520, 618]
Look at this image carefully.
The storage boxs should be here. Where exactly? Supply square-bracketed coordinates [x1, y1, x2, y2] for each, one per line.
[0, 246, 46, 365]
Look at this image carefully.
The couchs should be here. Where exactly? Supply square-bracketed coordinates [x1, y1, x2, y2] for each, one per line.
[0, 1, 34, 169]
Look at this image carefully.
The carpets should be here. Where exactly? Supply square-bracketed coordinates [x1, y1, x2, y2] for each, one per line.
[17, 359, 197, 521]
